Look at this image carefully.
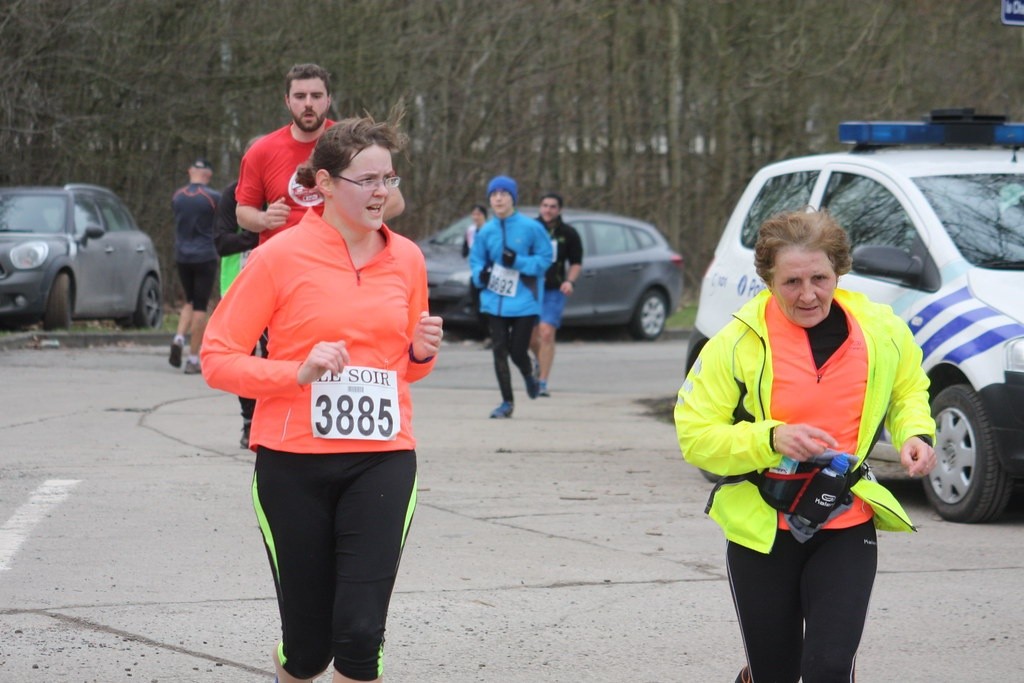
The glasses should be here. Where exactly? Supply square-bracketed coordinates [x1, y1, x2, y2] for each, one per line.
[332, 175, 400, 191]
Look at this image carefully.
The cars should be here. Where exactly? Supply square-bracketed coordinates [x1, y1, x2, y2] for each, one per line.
[415, 207, 684, 343]
[684, 108, 1023, 526]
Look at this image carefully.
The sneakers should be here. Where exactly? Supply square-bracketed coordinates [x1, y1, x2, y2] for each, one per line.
[538, 381, 549, 396]
[492, 402, 513, 417]
[169, 338, 183, 367]
[183, 361, 201, 374]
[525, 361, 538, 398]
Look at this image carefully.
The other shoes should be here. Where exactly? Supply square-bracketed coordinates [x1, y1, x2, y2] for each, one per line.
[240, 423, 249, 448]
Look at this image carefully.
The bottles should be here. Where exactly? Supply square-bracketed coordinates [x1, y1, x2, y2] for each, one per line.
[768, 453, 799, 475]
[791, 453, 850, 536]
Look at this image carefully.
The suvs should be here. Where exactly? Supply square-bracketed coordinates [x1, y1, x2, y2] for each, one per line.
[0, 184, 164, 330]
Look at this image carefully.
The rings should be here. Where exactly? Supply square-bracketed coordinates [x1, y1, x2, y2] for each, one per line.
[927, 465, 929, 468]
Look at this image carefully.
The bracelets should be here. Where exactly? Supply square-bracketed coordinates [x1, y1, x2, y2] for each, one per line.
[568, 280, 575, 288]
[774, 428, 776, 451]
[408, 342, 432, 364]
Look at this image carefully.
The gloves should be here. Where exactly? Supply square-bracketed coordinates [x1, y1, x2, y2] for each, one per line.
[479, 265, 492, 282]
[502, 247, 515, 267]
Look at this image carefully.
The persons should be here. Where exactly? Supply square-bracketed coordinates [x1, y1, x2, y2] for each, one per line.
[234, 63, 406, 246]
[528, 194, 583, 397]
[199, 99, 443, 683]
[673, 205, 936, 683]
[468, 176, 554, 419]
[461, 204, 494, 349]
[214, 135, 269, 449]
[169, 159, 222, 375]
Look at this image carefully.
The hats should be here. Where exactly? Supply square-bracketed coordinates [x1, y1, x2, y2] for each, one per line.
[485, 175, 517, 204]
